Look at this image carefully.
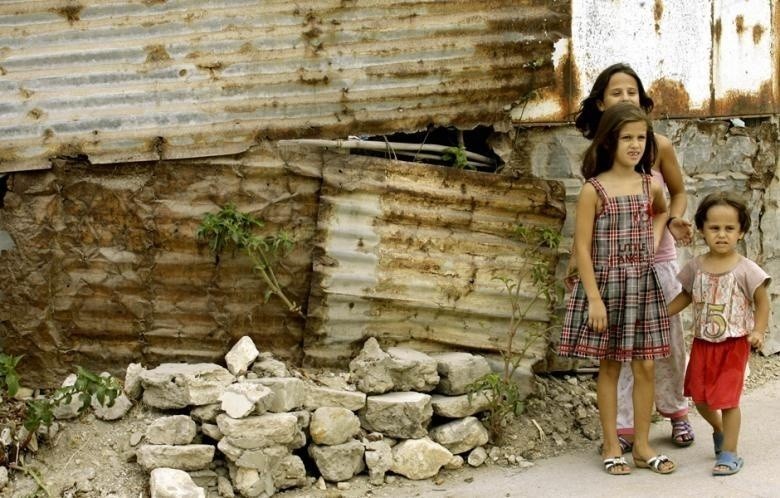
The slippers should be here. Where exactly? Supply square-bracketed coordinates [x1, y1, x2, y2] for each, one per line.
[671, 419, 695, 447]
[713, 451, 743, 476]
[632, 454, 677, 474]
[602, 457, 631, 475]
[598, 435, 633, 456]
[712, 430, 724, 458]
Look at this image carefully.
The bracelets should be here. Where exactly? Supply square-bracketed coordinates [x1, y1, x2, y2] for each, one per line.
[666, 216, 676, 229]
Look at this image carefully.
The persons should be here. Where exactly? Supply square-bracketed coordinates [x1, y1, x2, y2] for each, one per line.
[556, 100, 681, 476]
[563, 63, 697, 459]
[664, 190, 771, 477]
